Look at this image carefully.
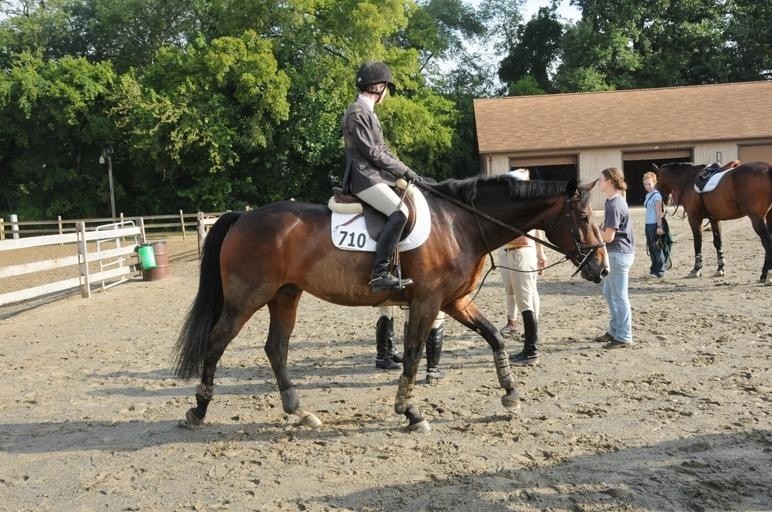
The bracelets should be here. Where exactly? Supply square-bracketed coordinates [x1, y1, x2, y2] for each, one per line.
[657, 223, 662, 228]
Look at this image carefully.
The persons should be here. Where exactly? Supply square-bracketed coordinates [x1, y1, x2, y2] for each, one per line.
[375, 167, 546, 385]
[340, 61, 414, 292]
[643, 171, 665, 277]
[595, 168, 635, 349]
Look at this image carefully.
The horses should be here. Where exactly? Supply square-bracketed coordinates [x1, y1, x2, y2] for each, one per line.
[652, 161, 772, 283]
[171, 174, 610, 435]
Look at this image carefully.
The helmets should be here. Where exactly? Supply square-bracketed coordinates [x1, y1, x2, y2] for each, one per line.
[356, 62, 395, 97]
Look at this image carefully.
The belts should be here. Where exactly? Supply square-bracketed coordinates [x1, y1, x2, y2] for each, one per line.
[505, 244, 528, 251]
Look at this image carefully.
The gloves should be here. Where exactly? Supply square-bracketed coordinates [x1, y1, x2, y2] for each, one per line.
[404, 168, 423, 184]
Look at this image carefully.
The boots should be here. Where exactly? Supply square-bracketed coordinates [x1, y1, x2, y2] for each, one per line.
[376, 316, 402, 369]
[501, 310, 539, 366]
[426, 322, 444, 383]
[370, 211, 414, 292]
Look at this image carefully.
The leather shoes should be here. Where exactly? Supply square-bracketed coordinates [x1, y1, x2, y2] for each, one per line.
[597, 334, 631, 349]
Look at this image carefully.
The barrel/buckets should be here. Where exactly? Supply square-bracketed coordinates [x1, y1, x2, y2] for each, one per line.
[137, 245, 157, 271]
[142, 240, 169, 281]
